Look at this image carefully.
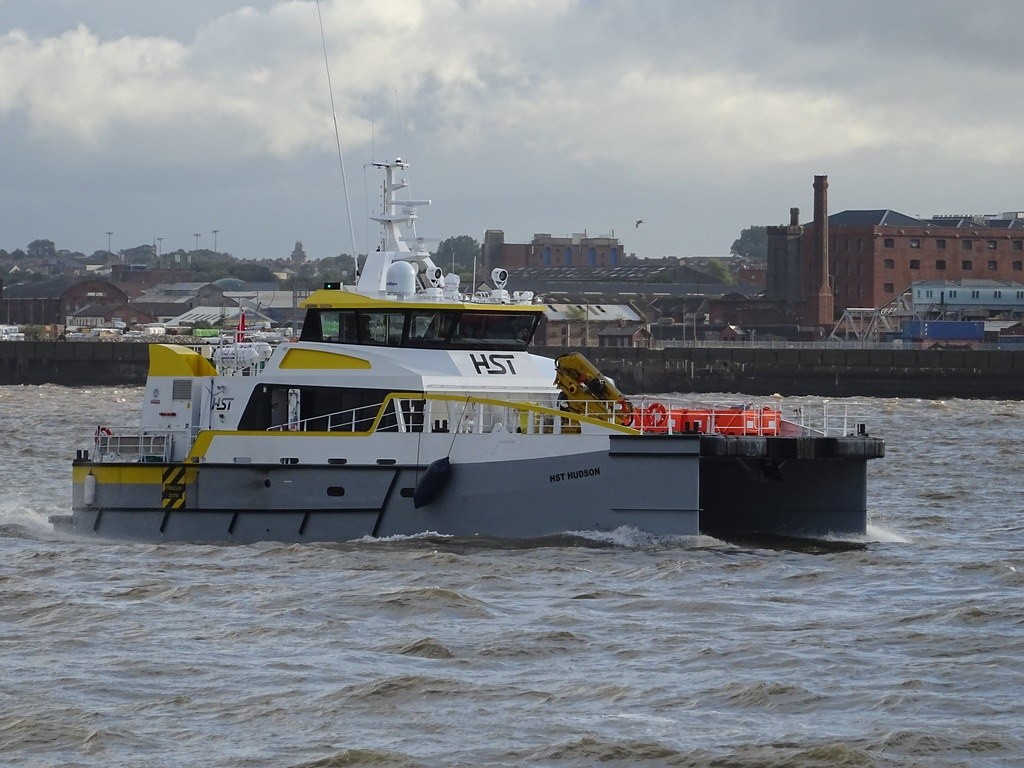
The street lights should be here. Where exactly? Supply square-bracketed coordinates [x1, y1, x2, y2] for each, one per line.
[212, 230, 219, 252]
[157, 238, 165, 256]
[194, 232, 201, 251]
[105, 231, 114, 284]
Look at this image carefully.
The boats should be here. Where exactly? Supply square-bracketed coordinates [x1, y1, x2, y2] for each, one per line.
[46, 2, 889, 555]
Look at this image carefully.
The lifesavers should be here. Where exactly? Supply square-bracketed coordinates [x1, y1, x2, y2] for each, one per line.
[647, 402, 667, 425]
[94, 426, 112, 445]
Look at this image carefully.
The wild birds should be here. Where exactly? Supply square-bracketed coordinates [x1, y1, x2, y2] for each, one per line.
[635, 220, 643, 228]
